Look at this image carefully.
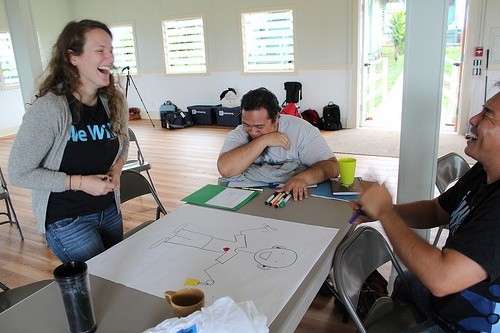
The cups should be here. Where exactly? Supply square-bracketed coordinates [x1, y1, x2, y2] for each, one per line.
[337, 157, 356, 184]
[163, 288, 206, 318]
[52, 261, 97, 333]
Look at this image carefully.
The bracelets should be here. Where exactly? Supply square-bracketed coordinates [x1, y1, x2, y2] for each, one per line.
[70, 175, 71, 190]
[76, 175, 82, 190]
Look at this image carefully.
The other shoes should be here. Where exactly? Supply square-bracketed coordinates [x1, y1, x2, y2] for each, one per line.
[319, 274, 334, 298]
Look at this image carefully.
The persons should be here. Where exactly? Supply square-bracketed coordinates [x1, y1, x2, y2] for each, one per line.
[217, 87, 340, 201]
[349, 92, 500, 333]
[6, 20, 129, 264]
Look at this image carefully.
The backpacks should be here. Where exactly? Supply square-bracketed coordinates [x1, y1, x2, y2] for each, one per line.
[281, 103, 300, 118]
[301, 110, 320, 129]
[322, 101, 342, 131]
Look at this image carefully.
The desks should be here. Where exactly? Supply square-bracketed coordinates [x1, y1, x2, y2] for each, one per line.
[0, 180, 370, 332]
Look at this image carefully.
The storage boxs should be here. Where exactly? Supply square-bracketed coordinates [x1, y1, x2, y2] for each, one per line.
[187, 104, 241, 126]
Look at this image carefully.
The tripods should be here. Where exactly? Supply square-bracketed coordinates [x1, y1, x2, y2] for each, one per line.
[121, 66, 155, 127]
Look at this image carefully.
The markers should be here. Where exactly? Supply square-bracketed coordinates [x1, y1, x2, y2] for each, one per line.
[269, 192, 282, 206]
[265, 192, 278, 205]
[280, 193, 292, 207]
[273, 192, 285, 206]
[348, 209, 361, 224]
[275, 194, 287, 208]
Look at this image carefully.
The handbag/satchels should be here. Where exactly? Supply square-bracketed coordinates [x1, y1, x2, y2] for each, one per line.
[168, 109, 188, 128]
[335, 269, 389, 324]
[284, 82, 302, 103]
[160, 100, 177, 128]
[128, 108, 141, 121]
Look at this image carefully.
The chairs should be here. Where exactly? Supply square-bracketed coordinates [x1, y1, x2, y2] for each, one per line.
[433, 153, 471, 247]
[120, 128, 167, 239]
[0, 167, 24, 241]
[0, 279, 55, 313]
[333, 227, 426, 333]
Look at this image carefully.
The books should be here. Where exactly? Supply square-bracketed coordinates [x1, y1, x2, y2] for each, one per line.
[329, 177, 363, 196]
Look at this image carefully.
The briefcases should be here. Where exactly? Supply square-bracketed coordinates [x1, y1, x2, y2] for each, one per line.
[187, 104, 222, 126]
[216, 87, 242, 127]
